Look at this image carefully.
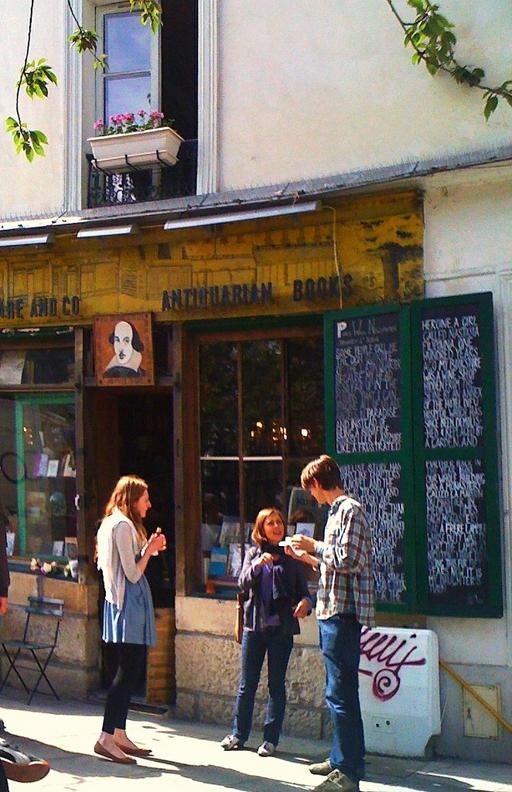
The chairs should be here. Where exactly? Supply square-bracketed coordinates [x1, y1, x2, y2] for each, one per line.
[1, 595, 67, 705]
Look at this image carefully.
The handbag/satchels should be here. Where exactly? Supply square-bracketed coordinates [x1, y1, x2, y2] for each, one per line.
[235, 591, 249, 643]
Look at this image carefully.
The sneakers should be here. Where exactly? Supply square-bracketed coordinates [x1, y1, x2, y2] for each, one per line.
[221, 735, 243, 750]
[0, 737, 49, 783]
[258, 741, 278, 756]
[308, 761, 333, 775]
[312, 769, 359, 792]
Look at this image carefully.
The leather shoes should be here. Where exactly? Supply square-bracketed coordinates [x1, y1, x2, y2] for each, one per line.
[116, 742, 152, 755]
[94, 741, 136, 764]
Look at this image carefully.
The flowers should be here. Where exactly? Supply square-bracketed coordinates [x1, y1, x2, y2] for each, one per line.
[92, 110, 177, 136]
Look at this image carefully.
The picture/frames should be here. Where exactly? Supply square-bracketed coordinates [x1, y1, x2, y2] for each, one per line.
[92, 311, 156, 388]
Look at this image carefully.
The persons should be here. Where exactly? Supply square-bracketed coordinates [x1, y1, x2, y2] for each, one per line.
[1, 527, 10, 615]
[101, 320, 148, 378]
[93, 475, 167, 765]
[220, 509, 309, 757]
[281, 458, 375, 792]
[1, 740, 52, 792]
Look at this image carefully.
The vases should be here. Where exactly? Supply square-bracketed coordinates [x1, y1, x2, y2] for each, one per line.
[85, 127, 183, 174]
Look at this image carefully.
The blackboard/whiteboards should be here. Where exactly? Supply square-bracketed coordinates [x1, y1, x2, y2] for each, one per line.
[410, 291, 505, 620]
[324, 304, 415, 614]
